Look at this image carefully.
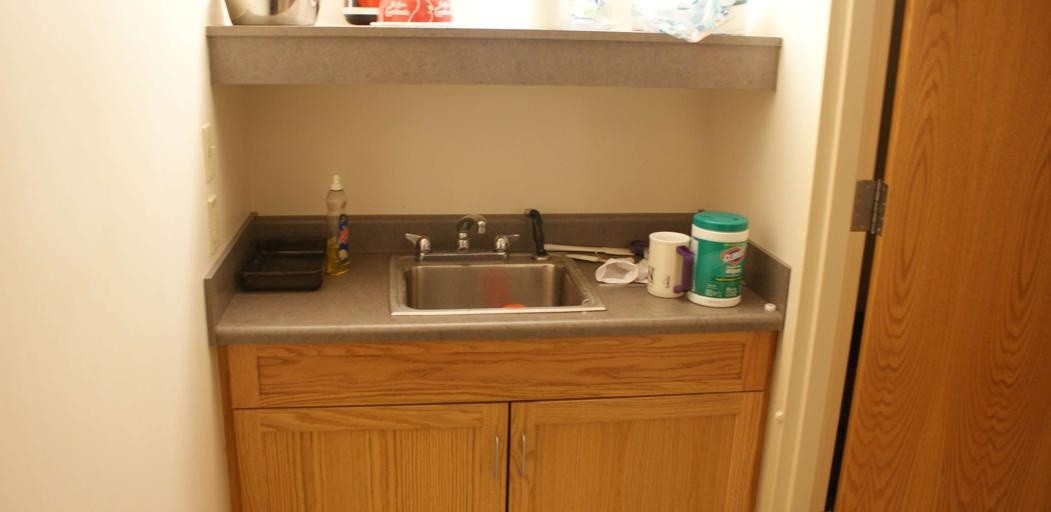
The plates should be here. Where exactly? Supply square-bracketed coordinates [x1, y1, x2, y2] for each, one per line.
[236, 236, 326, 291]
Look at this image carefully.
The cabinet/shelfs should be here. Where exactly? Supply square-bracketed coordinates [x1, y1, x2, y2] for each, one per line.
[218, 332, 779, 512]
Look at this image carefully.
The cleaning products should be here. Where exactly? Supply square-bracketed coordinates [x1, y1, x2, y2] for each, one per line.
[323, 174, 352, 277]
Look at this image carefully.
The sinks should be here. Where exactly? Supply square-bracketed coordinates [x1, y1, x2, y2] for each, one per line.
[389, 252, 607, 315]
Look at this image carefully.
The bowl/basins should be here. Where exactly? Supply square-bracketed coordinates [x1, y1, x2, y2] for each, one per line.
[224, 0, 319, 25]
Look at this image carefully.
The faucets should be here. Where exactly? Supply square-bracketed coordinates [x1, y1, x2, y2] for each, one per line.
[456, 215, 487, 254]
